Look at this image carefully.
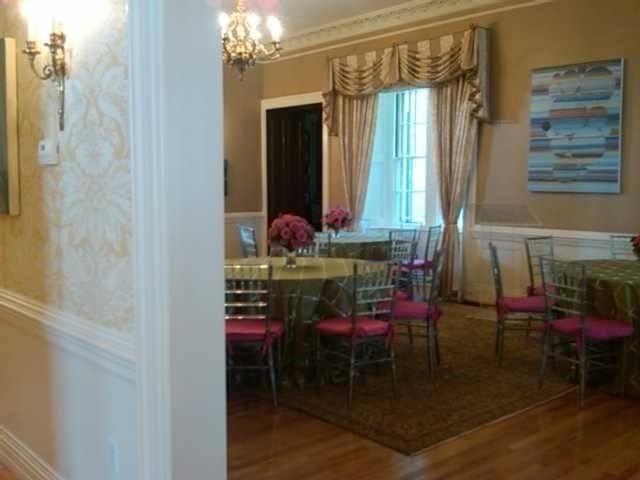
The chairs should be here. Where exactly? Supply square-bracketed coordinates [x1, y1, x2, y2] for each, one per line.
[524, 235, 566, 339]
[226, 262, 286, 408]
[548, 258, 640, 403]
[379, 247, 449, 379]
[237, 225, 446, 309]
[489, 242, 554, 367]
[310, 256, 402, 408]
[609, 233, 638, 259]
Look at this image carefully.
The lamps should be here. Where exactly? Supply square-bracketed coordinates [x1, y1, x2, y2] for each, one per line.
[218, 0, 283, 83]
[23, 14, 74, 132]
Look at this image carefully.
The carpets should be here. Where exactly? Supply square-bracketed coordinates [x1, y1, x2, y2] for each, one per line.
[278, 313, 580, 458]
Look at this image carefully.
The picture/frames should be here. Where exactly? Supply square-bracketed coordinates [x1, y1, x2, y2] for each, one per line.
[0, 36, 21, 216]
[526, 56, 624, 194]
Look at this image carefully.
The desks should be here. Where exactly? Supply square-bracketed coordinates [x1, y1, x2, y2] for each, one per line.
[536, 255, 633, 403]
[226, 256, 387, 385]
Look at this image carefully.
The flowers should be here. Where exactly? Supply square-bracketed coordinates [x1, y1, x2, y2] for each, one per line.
[266, 212, 317, 252]
[321, 206, 353, 228]
